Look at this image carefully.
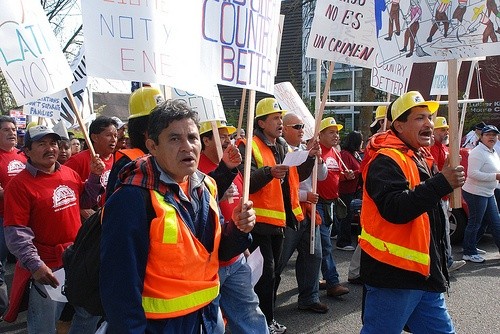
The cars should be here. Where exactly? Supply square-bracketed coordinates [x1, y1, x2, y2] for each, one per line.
[350, 147, 472, 246]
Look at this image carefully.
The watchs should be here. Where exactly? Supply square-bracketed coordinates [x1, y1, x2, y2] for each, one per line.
[322, 159, 324, 164]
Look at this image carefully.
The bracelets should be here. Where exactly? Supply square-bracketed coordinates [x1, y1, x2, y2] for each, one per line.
[343, 175, 348, 181]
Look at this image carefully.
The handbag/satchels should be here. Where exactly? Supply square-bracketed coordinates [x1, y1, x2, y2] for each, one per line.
[350, 199, 362, 222]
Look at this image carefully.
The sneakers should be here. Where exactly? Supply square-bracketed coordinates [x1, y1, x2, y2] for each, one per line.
[327, 285, 349, 295]
[447, 260, 466, 272]
[336, 242, 355, 250]
[463, 255, 487, 263]
[319, 280, 327, 291]
[330, 232, 337, 239]
[269, 319, 288, 334]
[298, 302, 330, 313]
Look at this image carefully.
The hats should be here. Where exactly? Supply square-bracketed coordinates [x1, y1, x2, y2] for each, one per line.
[476, 122, 487, 130]
[110, 117, 125, 130]
[483, 125, 500, 134]
[24, 125, 61, 145]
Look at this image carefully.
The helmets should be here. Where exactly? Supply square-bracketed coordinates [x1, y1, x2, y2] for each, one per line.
[254, 98, 287, 119]
[127, 87, 165, 120]
[433, 117, 449, 128]
[390, 91, 439, 126]
[318, 117, 343, 134]
[197, 120, 237, 134]
[374, 106, 388, 119]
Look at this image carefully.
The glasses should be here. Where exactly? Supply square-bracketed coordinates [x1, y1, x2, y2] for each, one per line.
[287, 124, 305, 130]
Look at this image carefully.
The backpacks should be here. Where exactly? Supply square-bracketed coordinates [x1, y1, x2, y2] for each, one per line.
[61, 181, 176, 316]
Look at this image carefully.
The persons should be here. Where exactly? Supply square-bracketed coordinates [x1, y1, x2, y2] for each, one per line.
[97, 97, 257, 334]
[0, 86, 500, 334]
[359, 90, 465, 334]
[3, 124, 106, 334]
[62, 118, 117, 226]
[461, 125, 500, 262]
[316, 117, 355, 298]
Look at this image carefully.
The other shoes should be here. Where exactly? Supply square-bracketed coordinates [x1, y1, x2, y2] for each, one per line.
[348, 276, 362, 284]
[462, 247, 486, 254]
